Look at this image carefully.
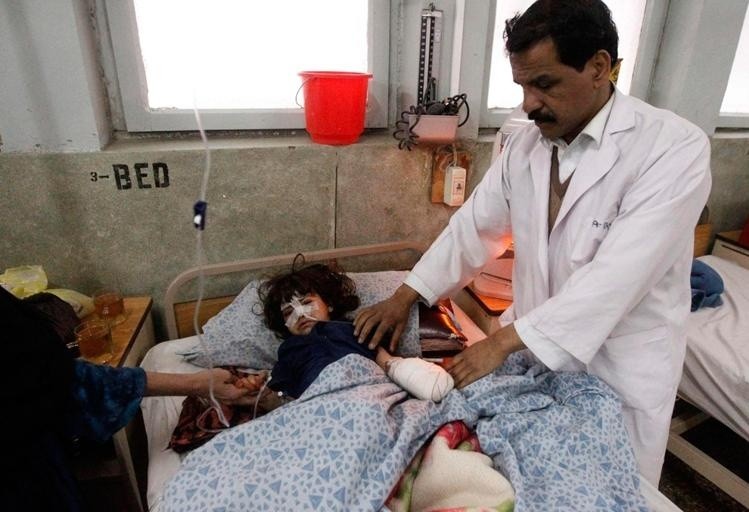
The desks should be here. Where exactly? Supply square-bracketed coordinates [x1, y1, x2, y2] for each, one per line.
[711, 230, 749, 269]
[463, 284, 513, 337]
[78, 298, 155, 512]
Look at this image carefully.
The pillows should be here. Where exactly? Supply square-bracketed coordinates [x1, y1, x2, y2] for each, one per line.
[420, 298, 467, 356]
[174, 270, 422, 368]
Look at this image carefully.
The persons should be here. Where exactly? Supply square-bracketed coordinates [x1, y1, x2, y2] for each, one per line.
[1, 284, 256, 512]
[225, 264, 454, 418]
[354, 1, 712, 488]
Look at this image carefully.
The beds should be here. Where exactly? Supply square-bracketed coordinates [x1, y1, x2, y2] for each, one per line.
[497, 222, 748, 508]
[138, 240, 683, 512]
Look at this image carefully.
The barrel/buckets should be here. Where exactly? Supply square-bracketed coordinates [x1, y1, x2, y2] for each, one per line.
[295, 70, 373, 145]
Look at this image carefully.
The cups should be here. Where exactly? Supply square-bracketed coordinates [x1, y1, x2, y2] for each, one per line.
[74, 324, 114, 365]
[94, 289, 127, 325]
